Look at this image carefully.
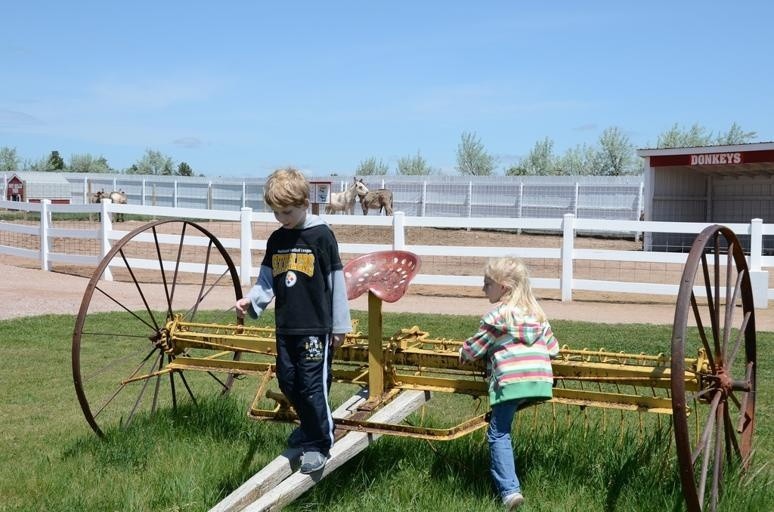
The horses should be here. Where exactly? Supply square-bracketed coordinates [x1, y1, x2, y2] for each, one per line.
[350, 176, 394, 216]
[89, 188, 127, 223]
[323, 179, 370, 215]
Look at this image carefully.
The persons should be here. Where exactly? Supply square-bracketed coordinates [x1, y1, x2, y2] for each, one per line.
[459, 256, 561, 511]
[234, 168, 354, 473]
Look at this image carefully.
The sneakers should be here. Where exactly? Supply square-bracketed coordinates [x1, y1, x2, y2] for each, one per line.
[299, 450, 328, 474]
[286, 425, 314, 449]
[503, 492, 525, 511]
